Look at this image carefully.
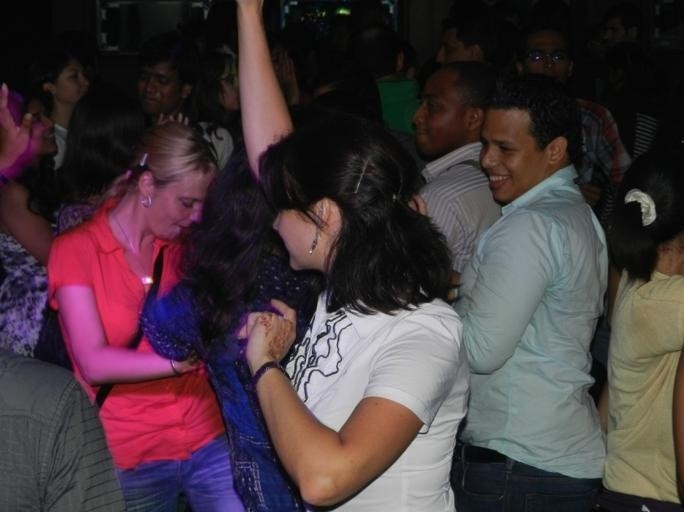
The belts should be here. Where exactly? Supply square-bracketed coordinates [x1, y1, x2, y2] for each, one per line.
[452, 442, 504, 465]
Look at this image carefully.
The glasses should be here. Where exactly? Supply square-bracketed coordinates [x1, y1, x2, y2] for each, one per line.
[522, 49, 571, 66]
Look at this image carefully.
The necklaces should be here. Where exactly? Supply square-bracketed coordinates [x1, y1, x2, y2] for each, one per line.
[113, 212, 153, 285]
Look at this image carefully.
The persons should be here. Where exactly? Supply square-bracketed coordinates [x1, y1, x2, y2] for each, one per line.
[237, 4, 471, 510]
[261, 4, 683, 452]
[453, 72, 608, 510]
[411, 60, 508, 273]
[601, 146, 682, 509]
[0, 4, 319, 509]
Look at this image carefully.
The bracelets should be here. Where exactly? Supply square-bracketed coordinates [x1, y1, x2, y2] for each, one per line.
[170, 360, 183, 375]
[252, 361, 291, 388]
